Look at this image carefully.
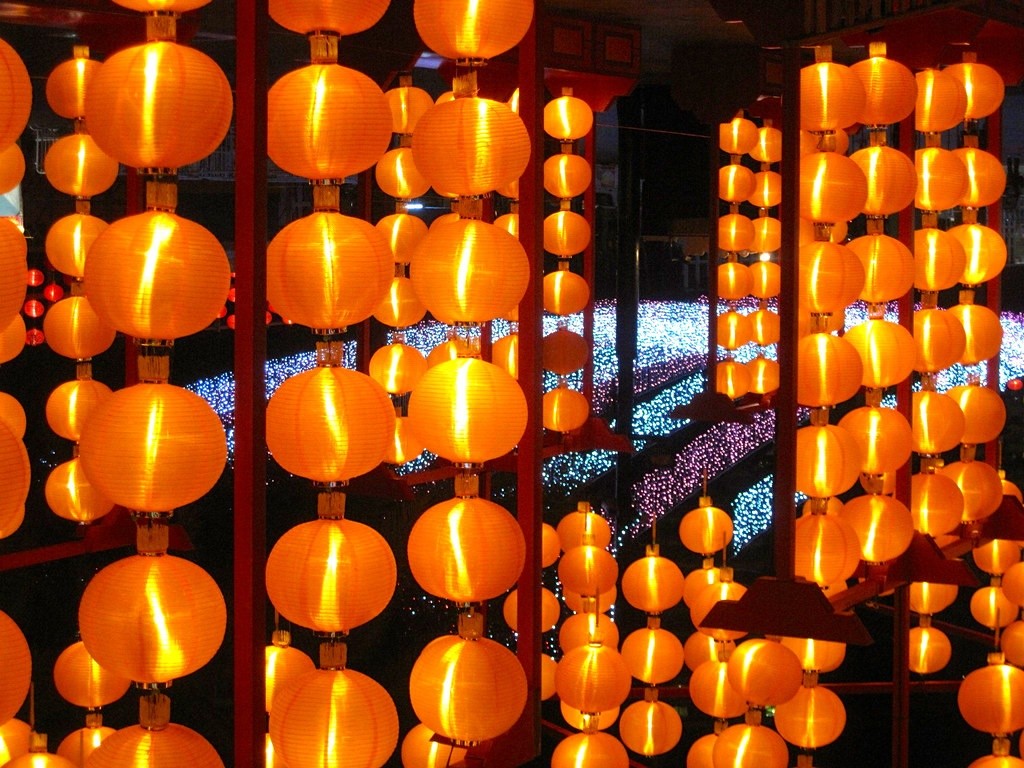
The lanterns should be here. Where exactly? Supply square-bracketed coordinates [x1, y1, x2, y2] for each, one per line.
[264, 0, 532, 768]
[0, 1, 234, 768]
[535, 44, 1024, 768]
